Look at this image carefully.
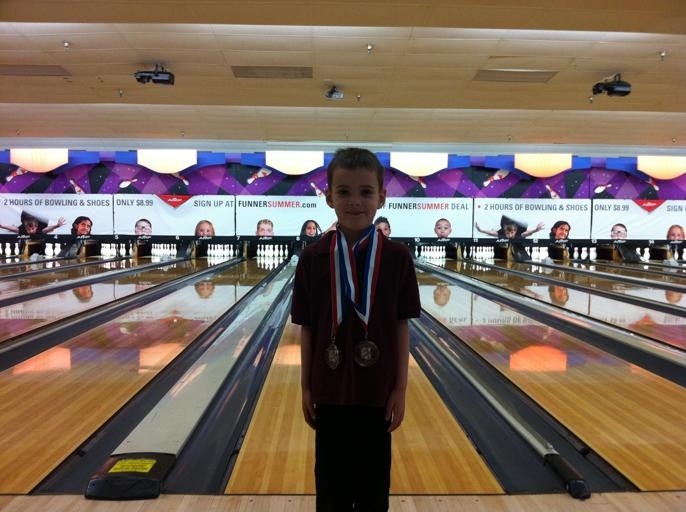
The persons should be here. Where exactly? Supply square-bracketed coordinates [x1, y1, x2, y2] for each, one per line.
[666, 283, 683, 304]
[195, 280, 215, 298]
[0, 212, 67, 234]
[475, 221, 543, 239]
[610, 223, 627, 239]
[195, 220, 215, 236]
[549, 283, 570, 306]
[291, 147, 421, 512]
[435, 218, 452, 238]
[373, 216, 391, 238]
[433, 283, 452, 306]
[300, 220, 338, 237]
[74, 284, 94, 302]
[256, 219, 274, 236]
[667, 225, 685, 241]
[549, 221, 571, 241]
[135, 219, 153, 235]
[70, 217, 94, 235]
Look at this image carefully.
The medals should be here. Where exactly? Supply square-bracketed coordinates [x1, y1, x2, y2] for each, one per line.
[353, 340, 380, 368]
[326, 345, 342, 370]
